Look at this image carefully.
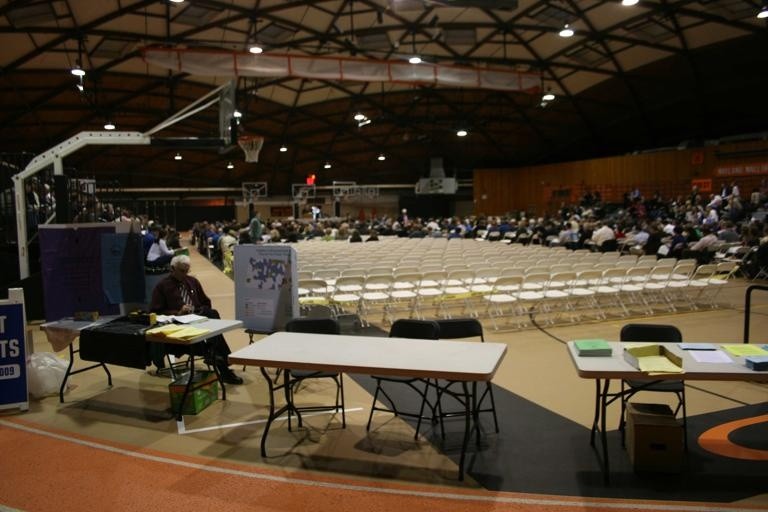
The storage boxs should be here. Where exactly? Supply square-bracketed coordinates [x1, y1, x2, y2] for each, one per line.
[625, 401, 676, 466]
[167, 370, 218, 418]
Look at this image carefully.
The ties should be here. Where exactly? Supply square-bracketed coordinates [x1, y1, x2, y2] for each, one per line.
[178, 283, 194, 313]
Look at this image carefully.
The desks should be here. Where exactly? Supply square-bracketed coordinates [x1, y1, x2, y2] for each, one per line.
[227, 330, 508, 481]
[567, 339, 767, 478]
[41, 312, 243, 422]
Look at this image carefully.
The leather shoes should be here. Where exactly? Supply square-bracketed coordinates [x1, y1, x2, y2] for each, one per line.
[220, 371, 243, 385]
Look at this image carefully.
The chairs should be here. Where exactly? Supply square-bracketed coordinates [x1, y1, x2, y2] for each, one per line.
[617, 322, 689, 447]
[366, 318, 445, 440]
[433, 317, 500, 441]
[281, 238, 741, 331]
[284, 317, 349, 432]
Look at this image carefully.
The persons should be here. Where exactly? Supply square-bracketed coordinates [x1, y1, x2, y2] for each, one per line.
[192, 212, 325, 272]
[316, 215, 535, 246]
[149, 255, 242, 385]
[24, 177, 181, 273]
[532, 181, 767, 265]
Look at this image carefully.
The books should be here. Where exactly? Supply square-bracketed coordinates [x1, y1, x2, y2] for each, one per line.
[575, 339, 611, 357]
[146, 313, 207, 341]
